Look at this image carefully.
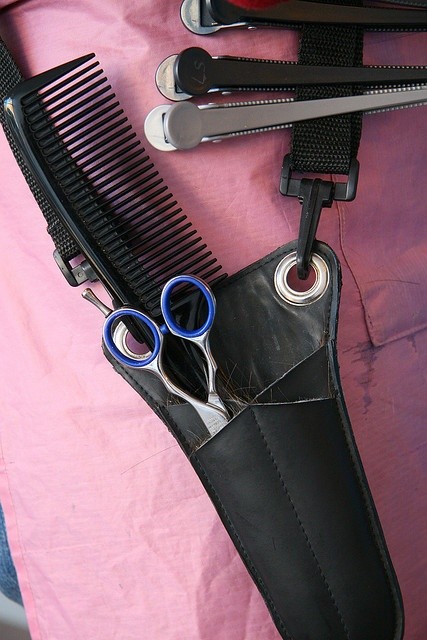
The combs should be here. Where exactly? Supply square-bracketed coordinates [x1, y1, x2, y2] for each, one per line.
[4, 54, 228, 403]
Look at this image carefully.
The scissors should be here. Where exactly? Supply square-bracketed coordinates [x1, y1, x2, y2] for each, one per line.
[101, 273, 231, 437]
